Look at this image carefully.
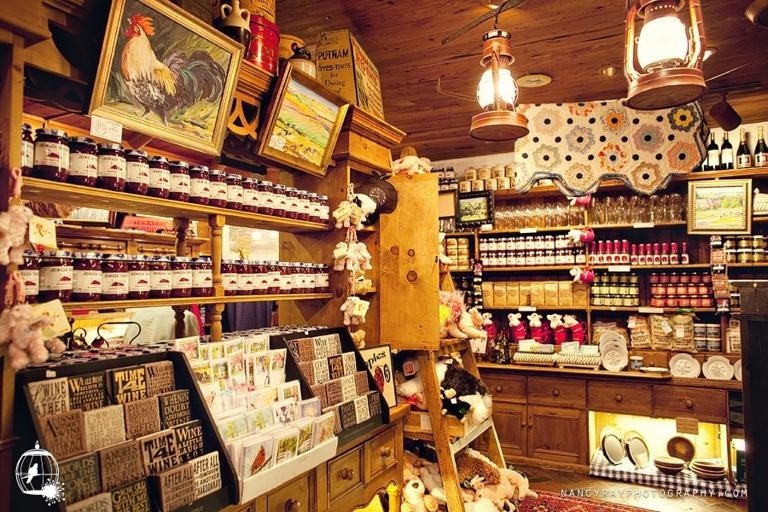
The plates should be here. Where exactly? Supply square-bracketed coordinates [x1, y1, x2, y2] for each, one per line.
[702, 355, 734, 380]
[668, 353, 701, 378]
[599, 330, 627, 372]
[734, 359, 742, 381]
[640, 367, 669, 372]
[690, 460, 727, 480]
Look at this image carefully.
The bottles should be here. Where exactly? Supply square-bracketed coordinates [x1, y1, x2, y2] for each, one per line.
[309, 193, 320, 222]
[763, 250, 768, 261]
[629, 283, 639, 295]
[258, 180, 274, 215]
[707, 131, 721, 171]
[605, 240, 613, 265]
[227, 174, 242, 209]
[128, 255, 151, 301]
[661, 242, 669, 264]
[237, 260, 256, 295]
[754, 126, 768, 167]
[697, 284, 709, 294]
[710, 242, 723, 263]
[670, 243, 678, 265]
[221, 261, 238, 296]
[653, 244, 661, 264]
[604, 295, 614, 306]
[544, 235, 555, 249]
[17, 253, 39, 303]
[645, 243, 654, 264]
[507, 238, 515, 251]
[713, 267, 725, 280]
[690, 294, 700, 307]
[209, 169, 227, 208]
[690, 272, 700, 283]
[432, 166, 458, 192]
[274, 184, 286, 217]
[650, 284, 656, 294]
[507, 252, 516, 266]
[97, 143, 125, 192]
[638, 243, 645, 265]
[565, 250, 574, 263]
[546, 250, 555, 264]
[693, 339, 706, 353]
[268, 261, 280, 294]
[654, 296, 665, 307]
[707, 324, 721, 338]
[515, 236, 524, 250]
[535, 250, 546, 265]
[452, 276, 475, 306]
[763, 236, 768, 250]
[650, 272, 660, 283]
[304, 262, 316, 293]
[729, 294, 740, 310]
[488, 253, 498, 266]
[192, 259, 213, 296]
[526, 236, 535, 250]
[753, 236, 763, 248]
[37, 128, 68, 183]
[592, 272, 601, 282]
[480, 252, 488, 267]
[284, 187, 299, 220]
[736, 127, 751, 168]
[660, 272, 669, 283]
[610, 272, 618, 283]
[720, 130, 733, 170]
[534, 235, 544, 249]
[315, 263, 330, 292]
[497, 238, 507, 251]
[622, 240, 629, 265]
[103, 254, 128, 300]
[656, 284, 665, 295]
[299, 190, 309, 221]
[73, 250, 104, 302]
[678, 295, 689, 307]
[280, 262, 292, 294]
[487, 238, 497, 251]
[737, 236, 753, 248]
[576, 249, 586, 264]
[702, 272, 711, 283]
[594, 295, 603, 305]
[479, 238, 487, 251]
[630, 244, 639, 265]
[740, 251, 752, 263]
[687, 283, 698, 294]
[564, 234, 574, 248]
[601, 272, 610, 283]
[575, 239, 582, 248]
[125, 149, 149, 196]
[319, 194, 330, 223]
[709, 284, 713, 294]
[722, 237, 737, 250]
[614, 296, 623, 306]
[169, 160, 190, 202]
[589, 241, 598, 265]
[679, 272, 690, 283]
[588, 195, 686, 228]
[597, 240, 606, 265]
[243, 176, 259, 213]
[667, 296, 678, 307]
[149, 156, 170, 199]
[669, 272, 679, 283]
[651, 295, 655, 306]
[68, 136, 97, 187]
[630, 272, 638, 283]
[613, 239, 621, 265]
[681, 242, 689, 264]
[624, 295, 632, 306]
[40, 251, 74, 303]
[170, 257, 192, 298]
[620, 283, 629, 294]
[632, 295, 639, 306]
[698, 295, 702, 307]
[516, 252, 526, 266]
[600, 283, 610, 294]
[497, 252, 506, 266]
[677, 284, 688, 295]
[20, 123, 34, 176]
[590, 283, 600, 294]
[555, 235, 564, 249]
[609, 283, 619, 294]
[702, 295, 712, 307]
[493, 203, 588, 233]
[756, 249, 764, 264]
[256, 260, 269, 295]
[707, 339, 721, 352]
[694, 324, 706, 338]
[663, 296, 667, 307]
[556, 250, 565, 263]
[726, 252, 736, 263]
[190, 165, 210, 205]
[291, 261, 304, 293]
[525, 251, 535, 265]
[620, 272, 629, 283]
[667, 284, 677, 295]
[151, 255, 171, 299]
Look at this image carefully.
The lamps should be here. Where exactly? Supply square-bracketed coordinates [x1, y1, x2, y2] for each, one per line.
[516, 74, 551, 89]
[622, 2, 708, 110]
[469, 0, 529, 142]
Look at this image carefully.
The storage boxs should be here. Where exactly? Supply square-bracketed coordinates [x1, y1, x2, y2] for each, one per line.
[317, 30, 386, 121]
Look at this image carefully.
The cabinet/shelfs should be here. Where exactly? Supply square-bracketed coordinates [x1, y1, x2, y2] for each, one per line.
[380, 165, 513, 512]
[1, 0, 407, 512]
[437, 166, 768, 499]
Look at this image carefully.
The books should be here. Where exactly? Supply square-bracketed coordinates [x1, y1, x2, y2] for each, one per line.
[174, 332, 335, 482]
[28, 359, 222, 512]
[289, 333, 381, 433]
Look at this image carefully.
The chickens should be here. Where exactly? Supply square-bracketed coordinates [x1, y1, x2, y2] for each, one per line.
[120, 12, 227, 127]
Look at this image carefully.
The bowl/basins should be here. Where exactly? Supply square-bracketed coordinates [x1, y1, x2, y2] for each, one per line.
[653, 456, 686, 476]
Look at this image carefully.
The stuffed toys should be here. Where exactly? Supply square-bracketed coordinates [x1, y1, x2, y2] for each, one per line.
[399, 436, 529, 512]
[334, 241, 373, 272]
[1, 206, 33, 266]
[336, 194, 375, 230]
[1, 303, 66, 371]
[348, 329, 365, 349]
[439, 288, 487, 340]
[392, 153, 453, 265]
[395, 353, 493, 427]
[341, 298, 366, 326]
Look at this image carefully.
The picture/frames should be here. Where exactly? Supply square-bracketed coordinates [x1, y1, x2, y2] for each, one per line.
[688, 180, 752, 237]
[255, 62, 350, 180]
[453, 189, 494, 229]
[83, 0, 246, 158]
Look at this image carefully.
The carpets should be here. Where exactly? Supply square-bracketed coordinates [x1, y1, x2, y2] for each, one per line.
[516, 490, 653, 512]
